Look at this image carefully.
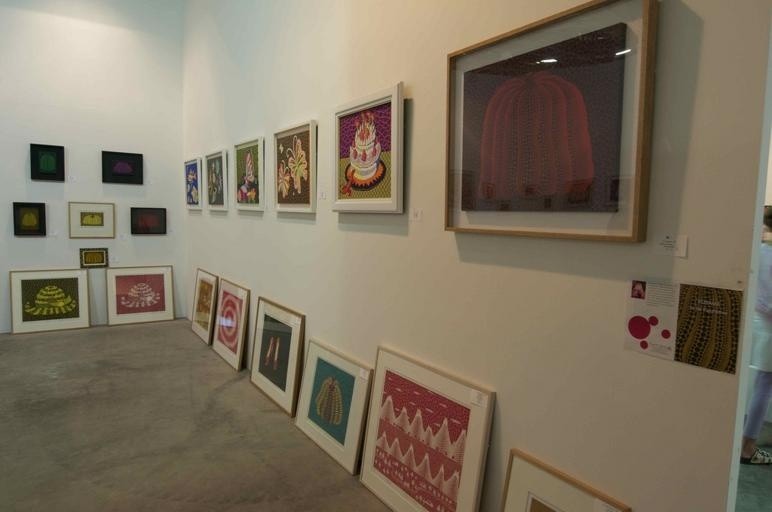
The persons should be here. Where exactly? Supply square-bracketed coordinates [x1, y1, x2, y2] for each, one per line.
[739, 205, 772, 467]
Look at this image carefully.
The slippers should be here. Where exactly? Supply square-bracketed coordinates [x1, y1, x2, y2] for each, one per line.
[741, 451, 772, 465]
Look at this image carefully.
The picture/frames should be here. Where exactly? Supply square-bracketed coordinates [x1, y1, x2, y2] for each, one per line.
[128, 206, 168, 236]
[443, 1, 659, 251]
[100, 149, 145, 187]
[29, 142, 66, 184]
[271, 117, 318, 218]
[79, 247, 109, 270]
[498, 446, 631, 511]
[12, 201, 48, 237]
[204, 147, 229, 214]
[232, 135, 266, 214]
[9, 267, 92, 336]
[182, 156, 203, 212]
[105, 265, 175, 327]
[330, 80, 408, 219]
[66, 199, 118, 240]
